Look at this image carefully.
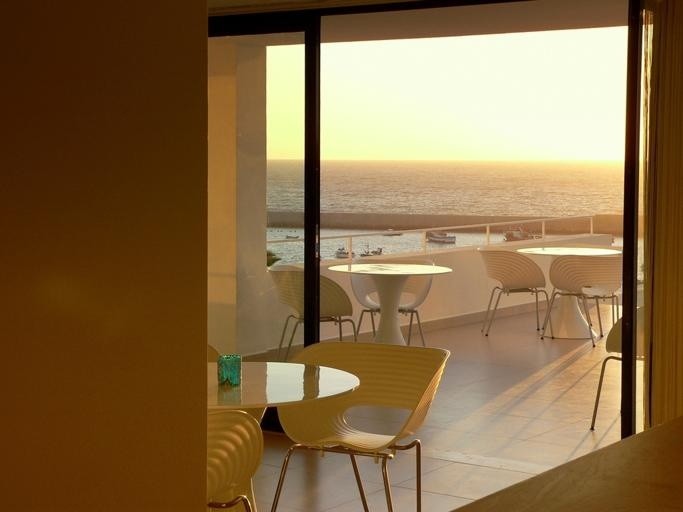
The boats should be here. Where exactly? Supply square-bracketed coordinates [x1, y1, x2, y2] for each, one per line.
[266, 251, 282, 269]
[503, 224, 542, 242]
[382, 228, 402, 237]
[359, 242, 382, 257]
[426, 230, 455, 244]
[335, 248, 353, 258]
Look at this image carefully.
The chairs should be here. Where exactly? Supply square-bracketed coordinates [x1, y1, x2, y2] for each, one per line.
[476, 244, 622, 348]
[589, 307, 644, 430]
